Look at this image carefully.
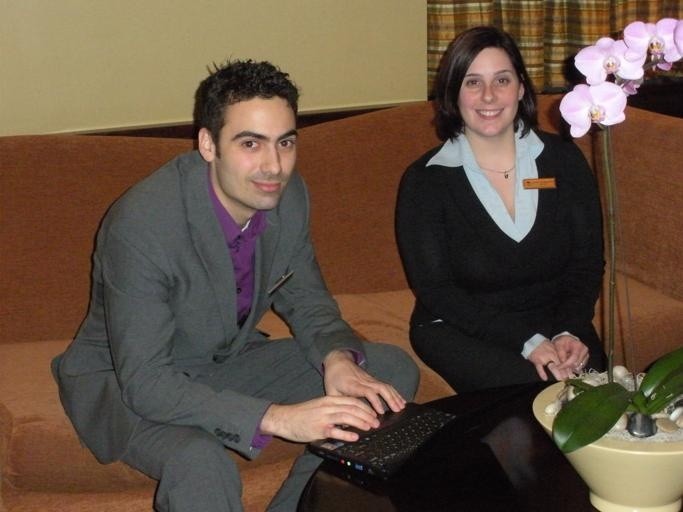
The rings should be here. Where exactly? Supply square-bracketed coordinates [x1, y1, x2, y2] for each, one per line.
[544, 359, 556, 367]
[579, 361, 585, 369]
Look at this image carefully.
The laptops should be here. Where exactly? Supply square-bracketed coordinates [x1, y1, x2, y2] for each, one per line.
[306, 388, 526, 494]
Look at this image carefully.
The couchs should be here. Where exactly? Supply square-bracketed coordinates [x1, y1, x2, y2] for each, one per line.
[0, 92, 682, 512]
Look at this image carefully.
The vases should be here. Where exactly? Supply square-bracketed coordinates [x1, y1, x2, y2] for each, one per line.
[528, 373, 682, 512]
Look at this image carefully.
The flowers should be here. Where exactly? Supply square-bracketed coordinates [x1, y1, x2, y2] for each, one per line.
[551, 12, 683, 454]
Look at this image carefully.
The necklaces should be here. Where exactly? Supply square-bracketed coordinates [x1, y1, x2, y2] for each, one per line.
[479, 165, 517, 179]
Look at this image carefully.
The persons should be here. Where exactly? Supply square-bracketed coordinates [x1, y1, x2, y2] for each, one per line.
[393, 26, 610, 396]
[47, 61, 422, 510]
